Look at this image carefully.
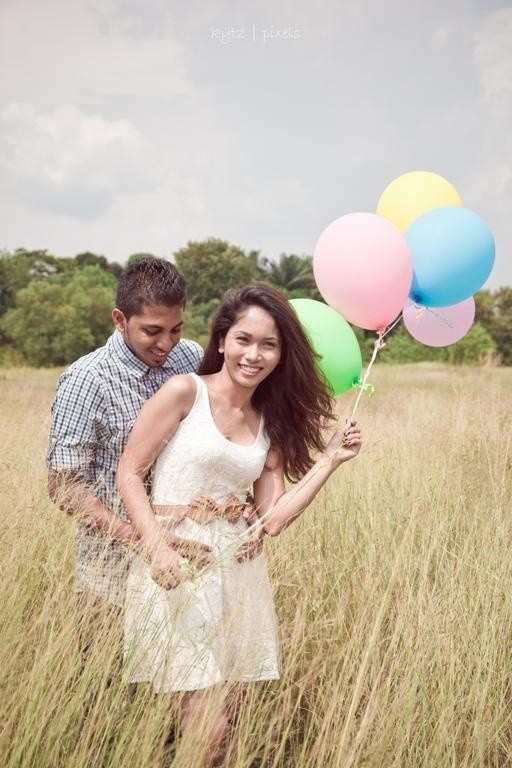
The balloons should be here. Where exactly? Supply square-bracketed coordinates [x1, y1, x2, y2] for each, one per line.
[307, 213, 412, 331]
[282, 291, 365, 394]
[401, 304, 479, 349]
[405, 206, 495, 310]
[375, 171, 462, 227]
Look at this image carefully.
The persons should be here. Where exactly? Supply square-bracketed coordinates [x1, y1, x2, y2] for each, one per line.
[50, 256, 207, 597]
[119, 286, 364, 767]
